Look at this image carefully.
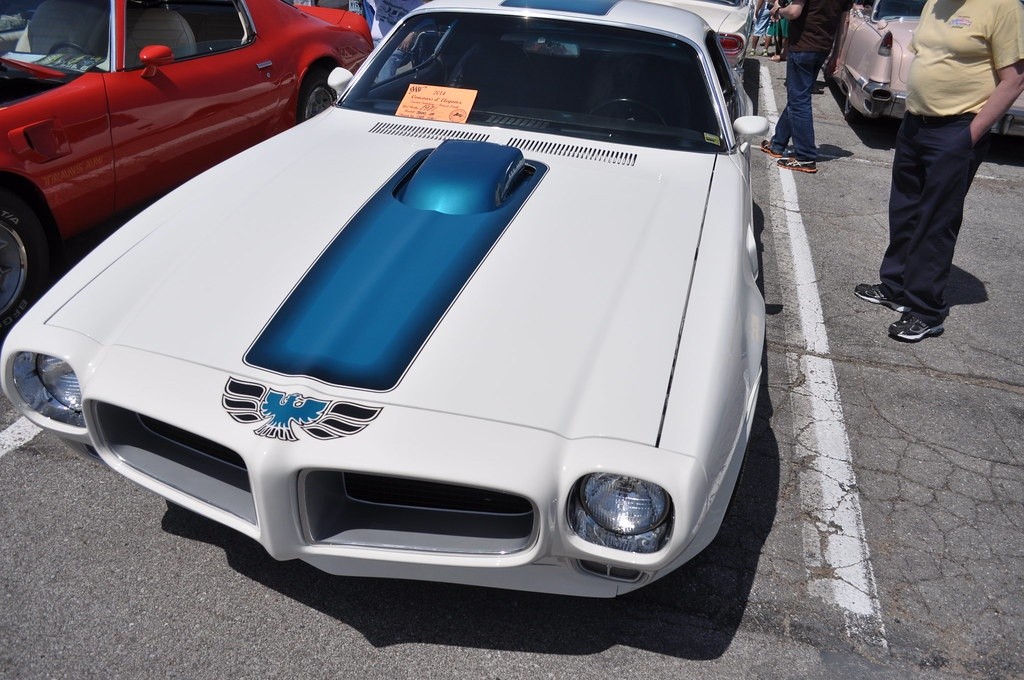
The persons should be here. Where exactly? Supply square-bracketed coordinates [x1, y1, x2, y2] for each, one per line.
[367, 0, 438, 85]
[759, 1, 851, 174]
[851, 0, 1024, 345]
[748, 1, 792, 62]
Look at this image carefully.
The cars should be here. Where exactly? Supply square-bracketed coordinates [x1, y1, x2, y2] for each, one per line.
[821, 0, 1024, 137]
[1, 0, 777, 602]
[1, 0, 376, 350]
[645, 1, 757, 71]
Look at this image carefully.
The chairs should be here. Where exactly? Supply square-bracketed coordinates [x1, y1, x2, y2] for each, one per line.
[14, 0, 108, 55]
[124, 8, 197, 69]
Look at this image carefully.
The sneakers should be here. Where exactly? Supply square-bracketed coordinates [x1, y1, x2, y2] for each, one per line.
[777, 158, 817, 173]
[888, 312, 945, 343]
[760, 139, 783, 158]
[854, 282, 912, 312]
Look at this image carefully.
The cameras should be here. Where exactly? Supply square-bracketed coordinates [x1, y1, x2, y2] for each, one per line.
[766, 0, 788, 19]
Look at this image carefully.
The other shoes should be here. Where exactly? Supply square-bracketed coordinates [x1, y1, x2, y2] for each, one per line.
[768, 54, 788, 61]
[749, 48, 755, 56]
[762, 49, 768, 57]
